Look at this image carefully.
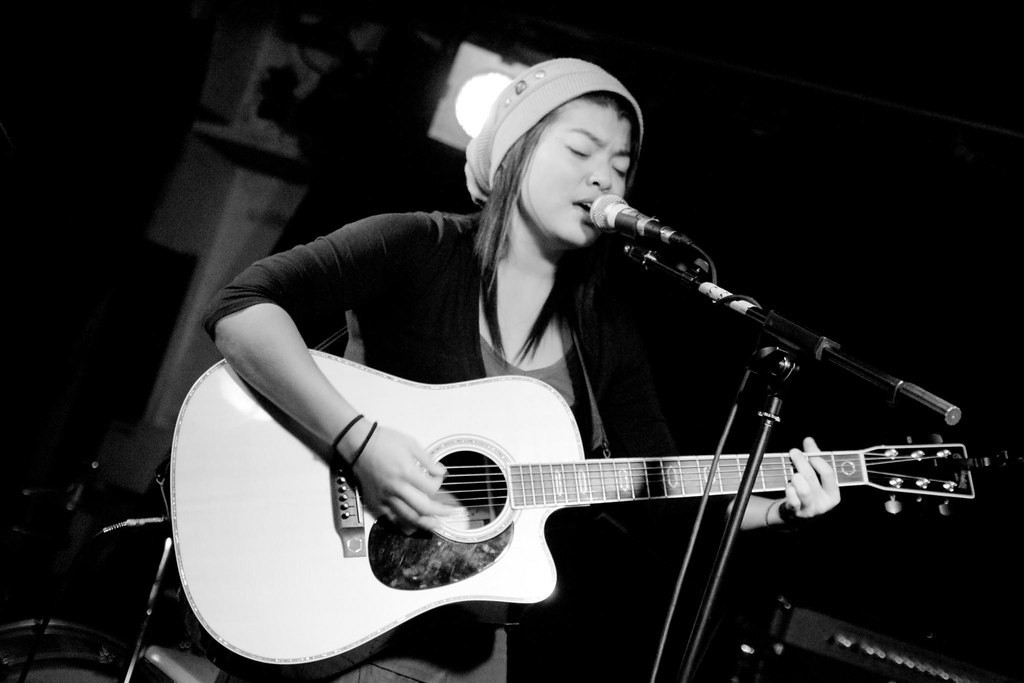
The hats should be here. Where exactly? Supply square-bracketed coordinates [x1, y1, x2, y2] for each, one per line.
[469, 57, 644, 212]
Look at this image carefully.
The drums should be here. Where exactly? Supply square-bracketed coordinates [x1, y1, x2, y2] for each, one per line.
[0, 617, 144, 683]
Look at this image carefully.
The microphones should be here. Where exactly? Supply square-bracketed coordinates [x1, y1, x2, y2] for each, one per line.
[590, 193, 693, 247]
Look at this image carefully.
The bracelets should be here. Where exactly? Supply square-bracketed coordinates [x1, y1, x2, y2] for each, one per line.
[348, 422, 378, 470]
[332, 415, 364, 450]
[766, 500, 778, 527]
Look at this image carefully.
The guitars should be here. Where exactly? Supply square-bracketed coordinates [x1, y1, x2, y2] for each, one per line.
[166, 343, 1024, 683]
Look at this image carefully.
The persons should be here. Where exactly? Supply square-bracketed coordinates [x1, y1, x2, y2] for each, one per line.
[202, 57, 842, 683]
